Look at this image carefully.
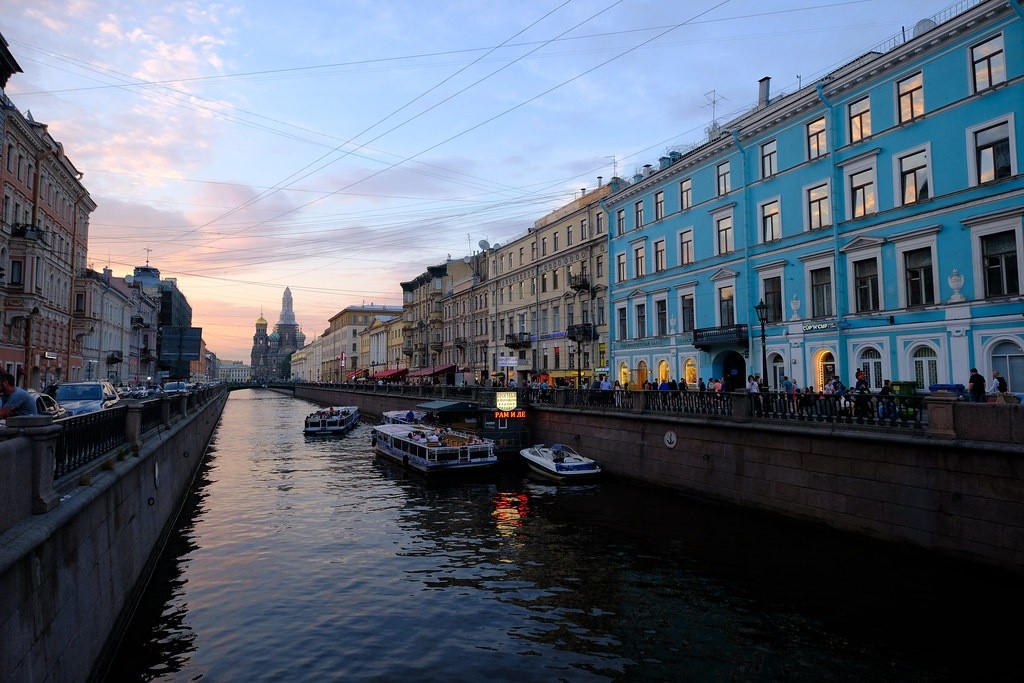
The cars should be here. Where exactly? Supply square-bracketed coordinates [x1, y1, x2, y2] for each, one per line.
[24, 388, 69, 430]
[112, 376, 219, 401]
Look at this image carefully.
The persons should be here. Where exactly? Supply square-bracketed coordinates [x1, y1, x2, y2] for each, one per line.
[304, 367, 1008, 446]
[0, 374, 38, 420]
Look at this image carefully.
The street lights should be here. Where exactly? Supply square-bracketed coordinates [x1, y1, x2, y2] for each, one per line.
[395, 357, 400, 386]
[575, 328, 584, 404]
[482, 343, 488, 379]
[752, 299, 770, 411]
[371, 360, 376, 384]
[431, 352, 436, 386]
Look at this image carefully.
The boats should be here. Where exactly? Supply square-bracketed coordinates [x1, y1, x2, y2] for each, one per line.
[372, 424, 499, 473]
[519, 442, 601, 483]
[418, 399, 531, 452]
[381, 409, 440, 424]
[303, 406, 360, 433]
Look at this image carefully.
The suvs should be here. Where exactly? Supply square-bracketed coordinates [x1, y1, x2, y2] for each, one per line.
[42, 380, 122, 424]
